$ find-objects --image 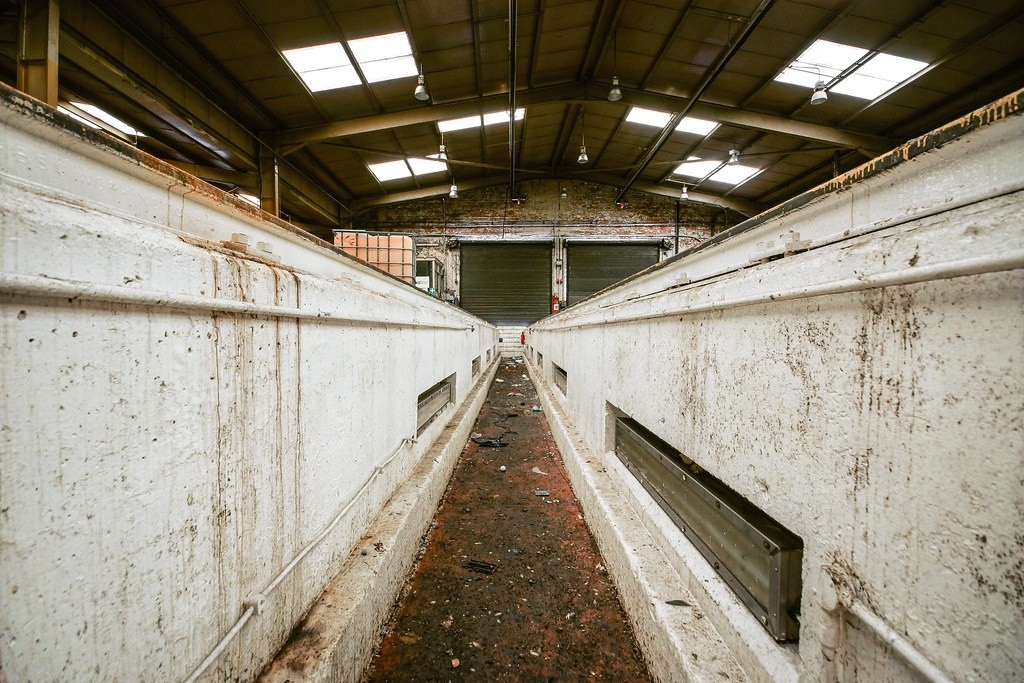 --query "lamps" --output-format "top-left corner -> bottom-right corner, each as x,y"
811,68 -> 828,105
449,177 -> 458,198
560,185 -> 568,199
577,113 -> 588,163
438,133 -> 447,162
607,31 -> 622,101
729,148 -> 740,163
680,181 -> 688,200
414,62 -> 429,100
